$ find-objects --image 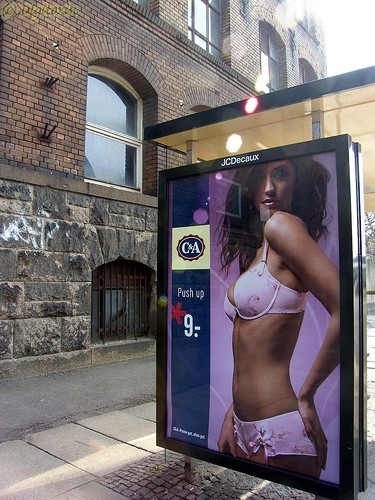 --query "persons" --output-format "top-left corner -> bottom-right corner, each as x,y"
217,152 -> 339,480
